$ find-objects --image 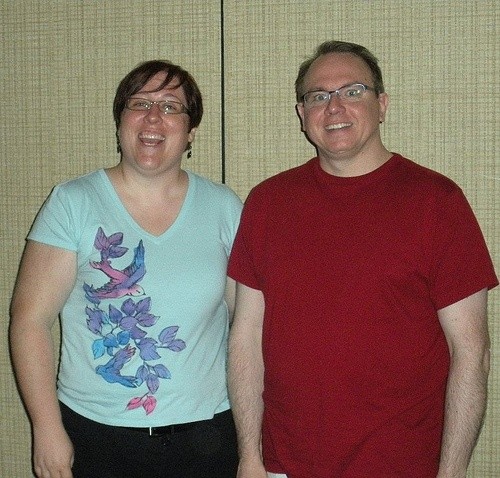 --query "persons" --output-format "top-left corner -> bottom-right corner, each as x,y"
225,39 -> 500,477
8,58 -> 243,478
